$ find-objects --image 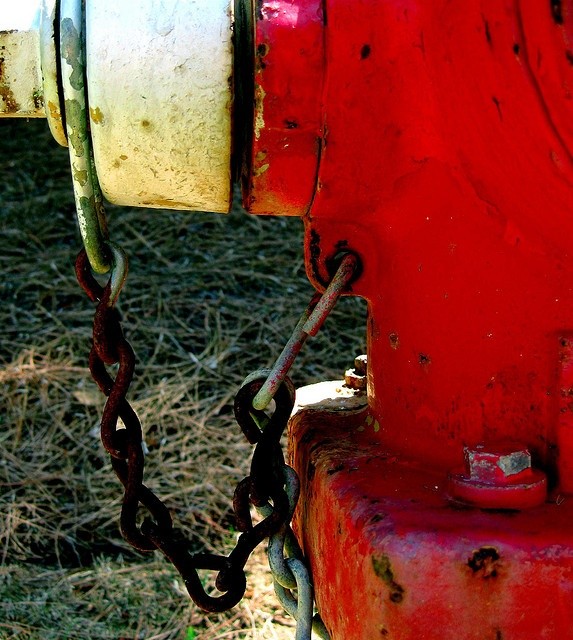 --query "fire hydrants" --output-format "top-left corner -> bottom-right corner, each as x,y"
2,0 -> 571,640
